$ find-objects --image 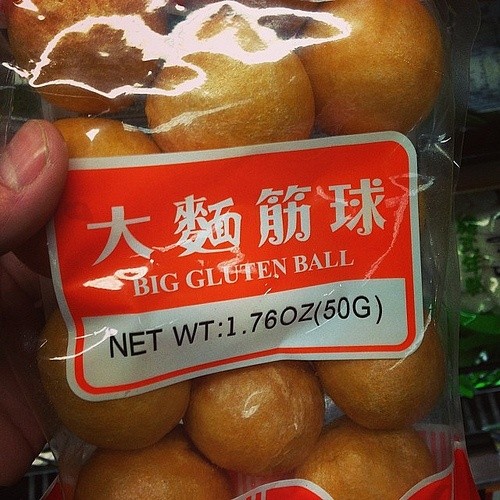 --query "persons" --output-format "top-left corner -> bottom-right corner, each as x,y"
0,117 -> 77,487
